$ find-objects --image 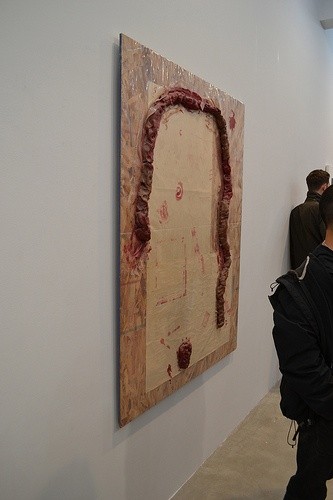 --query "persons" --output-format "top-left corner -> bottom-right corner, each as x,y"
263,185 -> 333,500
287,168 -> 329,269
